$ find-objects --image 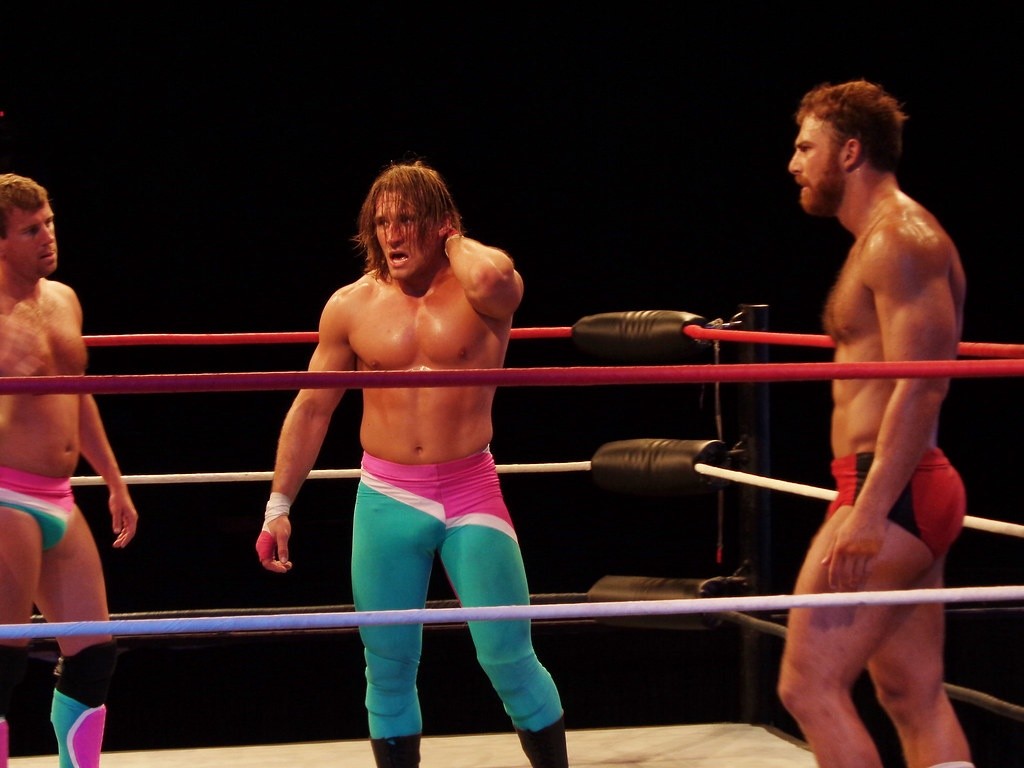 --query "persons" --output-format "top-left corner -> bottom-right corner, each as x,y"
255,164 -> 568,768
776,79 -> 971,768
0,173 -> 138,768
